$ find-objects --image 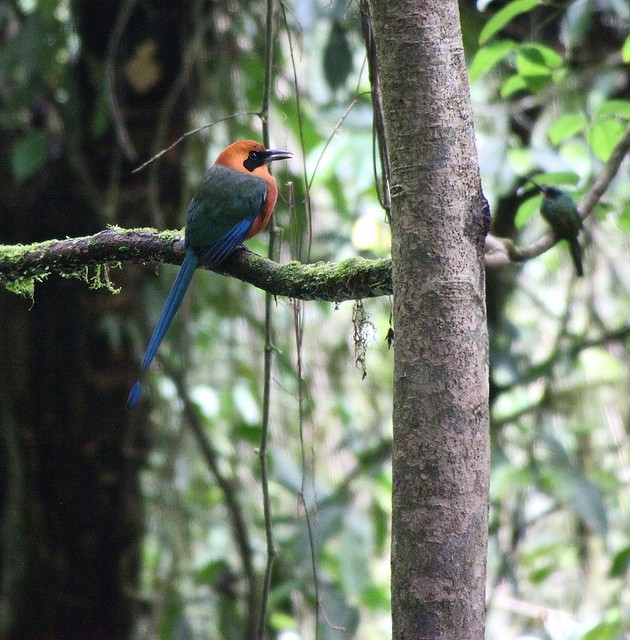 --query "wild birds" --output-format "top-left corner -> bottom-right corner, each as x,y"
523,175 -> 587,279
122,138 -> 297,413
